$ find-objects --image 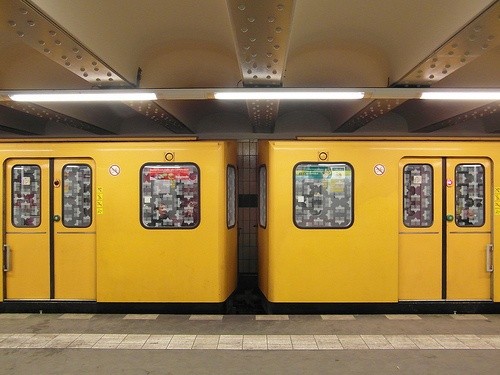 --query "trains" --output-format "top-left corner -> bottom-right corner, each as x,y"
0,135 -> 500,316
